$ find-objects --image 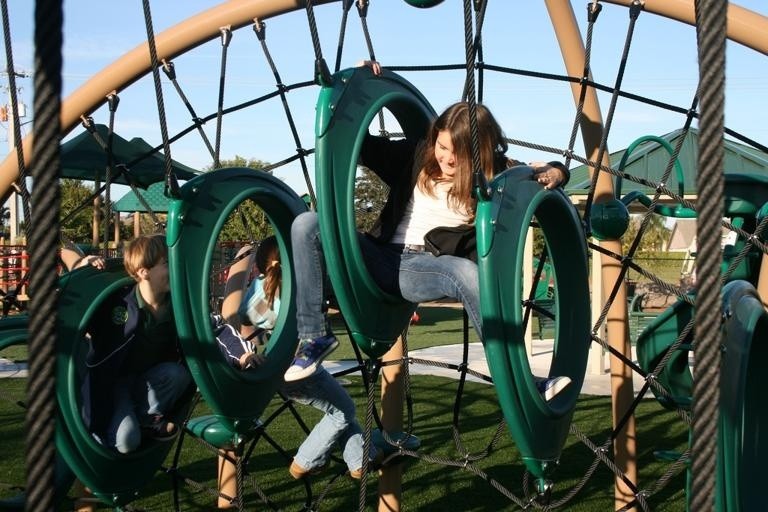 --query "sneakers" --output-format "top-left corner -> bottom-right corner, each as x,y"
140,421 -> 179,442
283,333 -> 341,382
349,446 -> 383,478
536,376 -> 572,402
288,456 -> 330,480
